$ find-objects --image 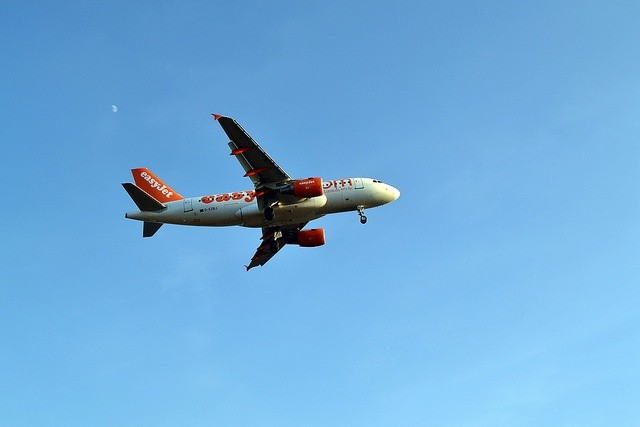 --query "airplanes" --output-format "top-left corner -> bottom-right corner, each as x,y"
121,113 -> 401,271
